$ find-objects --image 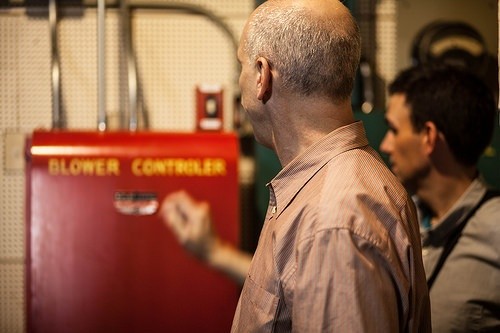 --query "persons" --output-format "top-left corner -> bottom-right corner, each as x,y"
159,2 -> 434,333
380,60 -> 500,332
